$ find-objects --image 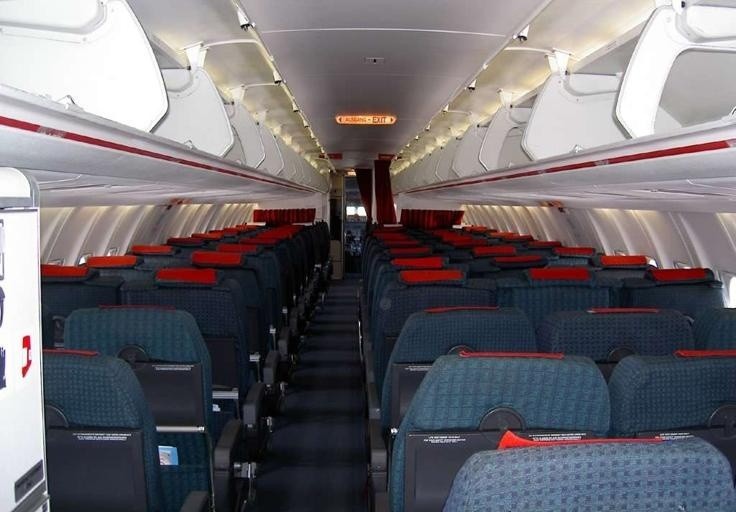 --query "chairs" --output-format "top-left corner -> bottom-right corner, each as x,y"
41,220 -> 332,511
357,226 -> 736,512
